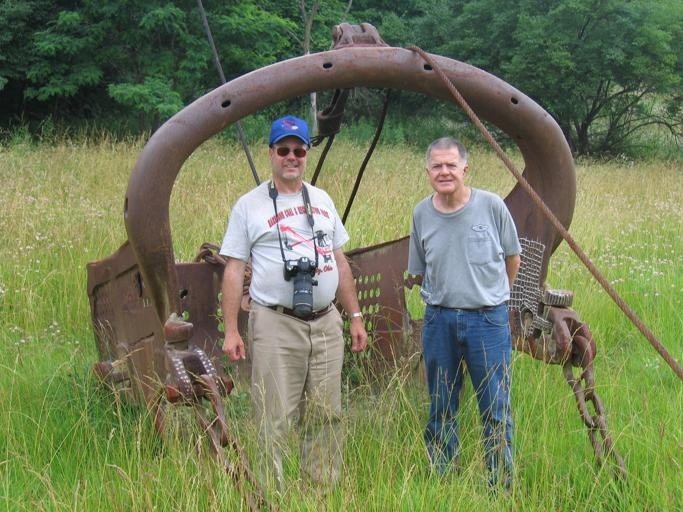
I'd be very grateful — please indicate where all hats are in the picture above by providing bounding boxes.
[268,116,310,146]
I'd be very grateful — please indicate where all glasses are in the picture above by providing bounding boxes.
[275,147,307,158]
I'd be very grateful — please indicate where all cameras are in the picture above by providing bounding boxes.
[282,259,320,316]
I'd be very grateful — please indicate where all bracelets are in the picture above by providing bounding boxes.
[347,311,364,321]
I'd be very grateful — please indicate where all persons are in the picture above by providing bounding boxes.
[217,115,369,496]
[405,136,524,502]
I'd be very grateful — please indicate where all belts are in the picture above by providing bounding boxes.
[269,303,334,321]
